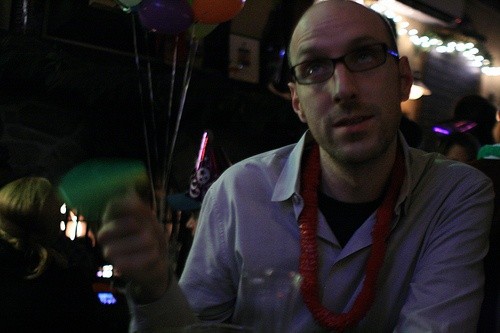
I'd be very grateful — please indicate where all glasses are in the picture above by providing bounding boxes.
[289,42,401,85]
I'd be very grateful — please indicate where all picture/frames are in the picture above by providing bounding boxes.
[226,32,264,87]
